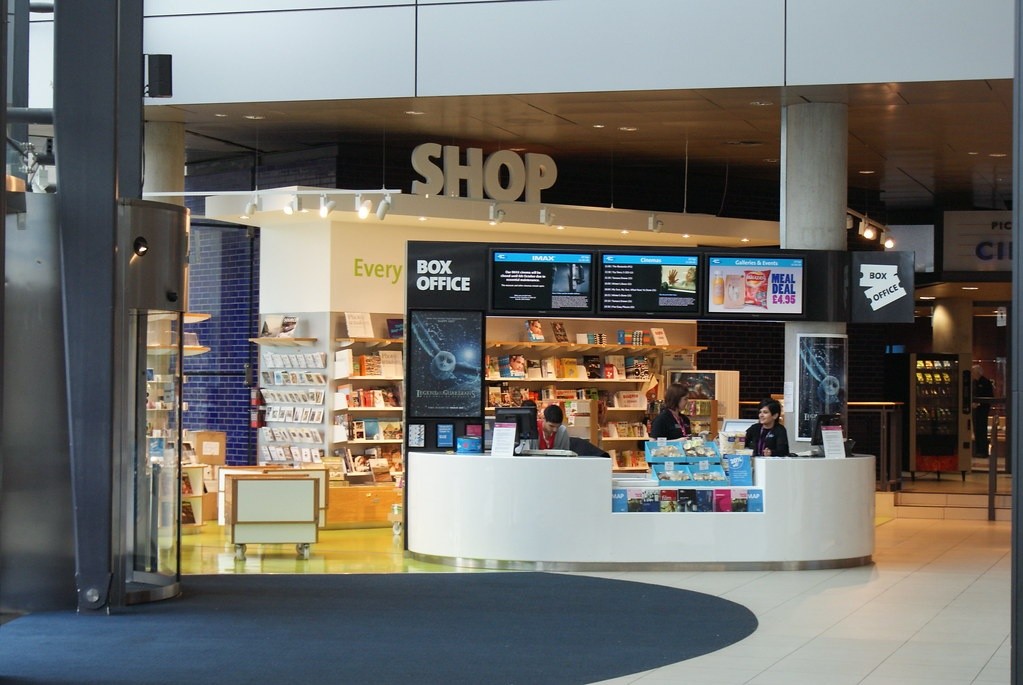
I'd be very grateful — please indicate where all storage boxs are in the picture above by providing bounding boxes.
[184,429,228,522]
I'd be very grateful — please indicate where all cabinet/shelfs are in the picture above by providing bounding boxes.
[157,463,208,534]
[326,331,406,534]
[479,313,697,474]
[666,368,741,450]
[133,309,213,529]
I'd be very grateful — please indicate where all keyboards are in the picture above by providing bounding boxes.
[521,449,578,457]
[795,449,818,457]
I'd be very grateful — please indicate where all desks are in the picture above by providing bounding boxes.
[217,463,329,544]
[219,472,319,561]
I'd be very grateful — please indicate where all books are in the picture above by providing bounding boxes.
[485,316,762,513]
[253,317,402,481]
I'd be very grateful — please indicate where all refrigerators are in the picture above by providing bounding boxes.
[909,351,972,475]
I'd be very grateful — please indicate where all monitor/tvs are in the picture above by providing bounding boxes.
[721,419,760,432]
[810,414,841,446]
[703,252,807,318]
[495,406,538,456]
[597,250,703,317]
[488,248,595,315]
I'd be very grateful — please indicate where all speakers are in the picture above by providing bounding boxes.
[148,54,172,97]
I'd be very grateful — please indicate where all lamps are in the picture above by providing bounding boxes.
[646,213,663,233]
[241,189,394,221]
[537,206,556,229]
[842,213,898,249]
[487,203,503,226]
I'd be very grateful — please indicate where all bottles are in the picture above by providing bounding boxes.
[711,269,724,305]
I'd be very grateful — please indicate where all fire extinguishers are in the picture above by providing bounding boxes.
[250,387,269,429]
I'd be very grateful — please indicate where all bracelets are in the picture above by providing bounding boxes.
[977,403,981,408]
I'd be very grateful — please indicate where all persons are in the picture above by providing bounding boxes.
[650,384,693,440]
[520,399,540,418]
[745,398,790,456]
[536,405,573,450]
[668,267,697,288]
[971,364,995,459]
[528,319,542,336]
[509,354,525,374]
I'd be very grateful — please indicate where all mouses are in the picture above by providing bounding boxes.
[787,453,798,457]
